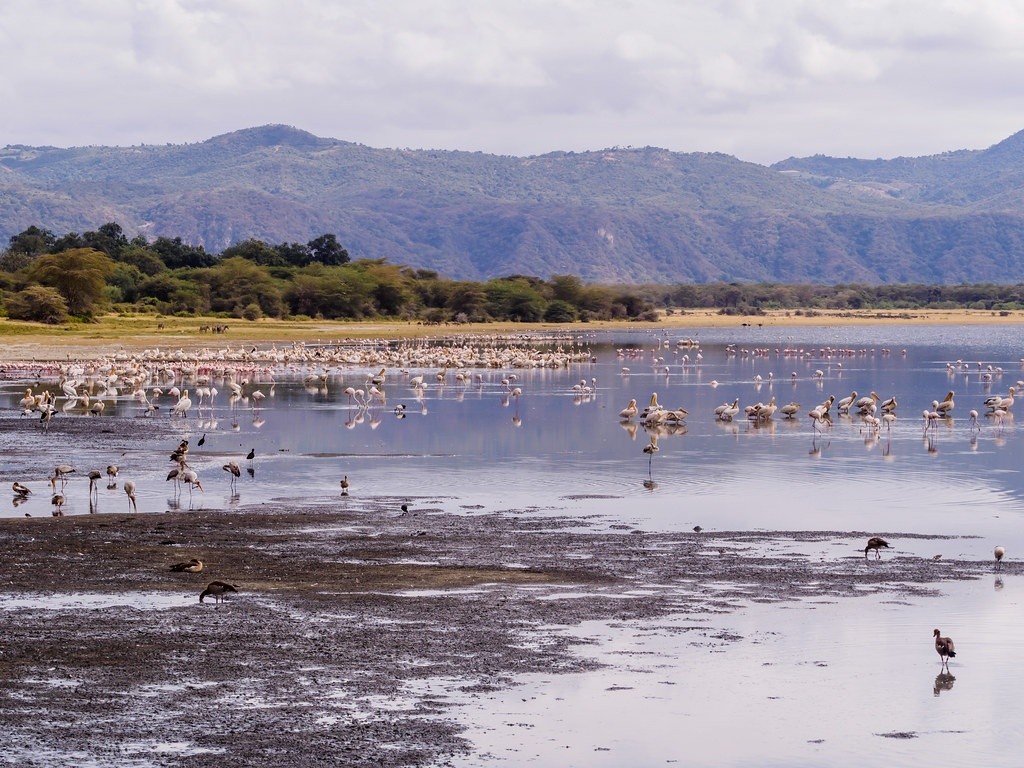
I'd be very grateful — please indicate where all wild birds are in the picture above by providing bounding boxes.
[0,330,1024,467]
[106,465,119,480]
[341,475,348,490]
[124,480,136,498]
[166,460,204,495]
[223,461,241,481]
[933,629,956,662]
[169,558,203,573]
[994,545,1004,561]
[199,581,238,604]
[88,470,102,488]
[12,482,33,495]
[52,495,64,507]
[865,538,890,559]
[52,465,76,487]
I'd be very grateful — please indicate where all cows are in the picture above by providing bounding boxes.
[200,323,229,333]
[417,320,471,326]
